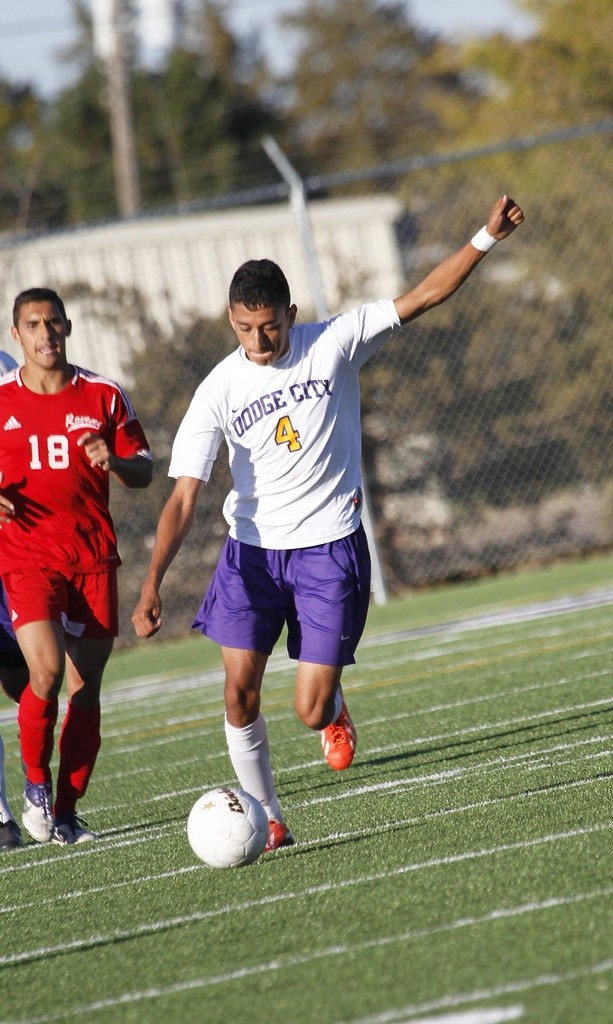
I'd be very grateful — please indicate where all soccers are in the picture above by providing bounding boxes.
[187,787,270,870]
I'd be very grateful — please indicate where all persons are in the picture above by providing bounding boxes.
[0,287,154,847]
[132,191,525,853]
[0,350,68,851]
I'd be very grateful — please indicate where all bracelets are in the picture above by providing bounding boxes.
[470,225,498,252]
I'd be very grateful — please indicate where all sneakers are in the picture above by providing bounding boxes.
[320,682,357,771]
[21,778,54,843]
[47,813,98,848]
[0,820,23,850]
[263,820,294,851]
